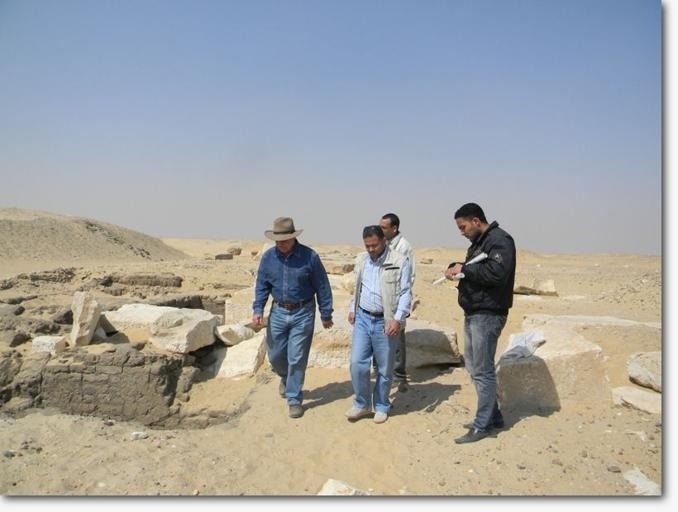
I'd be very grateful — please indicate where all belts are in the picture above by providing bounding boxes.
[358,304,384,317]
[274,296,314,310]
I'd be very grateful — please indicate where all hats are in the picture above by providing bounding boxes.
[263,216,305,242]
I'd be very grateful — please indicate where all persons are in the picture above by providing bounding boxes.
[250,217,336,420]
[442,202,518,445]
[377,213,417,394]
[344,224,413,425]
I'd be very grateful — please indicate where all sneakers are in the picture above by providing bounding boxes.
[287,403,304,418]
[372,409,389,423]
[278,377,289,398]
[345,402,373,420]
[397,378,409,393]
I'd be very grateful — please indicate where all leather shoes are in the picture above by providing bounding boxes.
[463,413,505,429]
[454,426,490,443]
